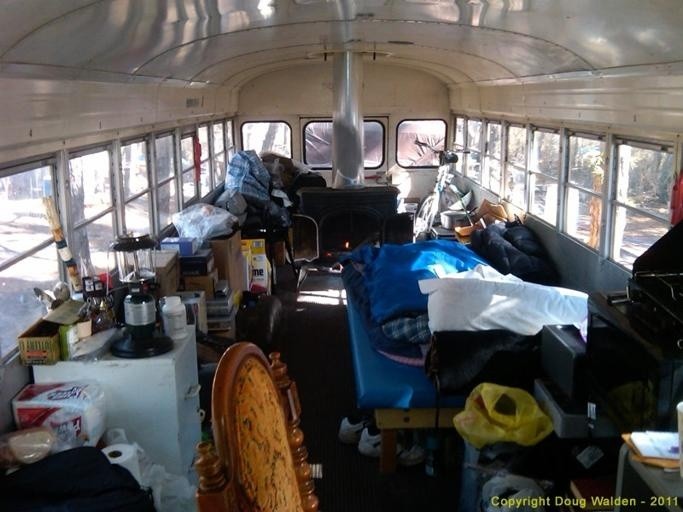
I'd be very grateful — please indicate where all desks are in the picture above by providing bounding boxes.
[614,430,682,512]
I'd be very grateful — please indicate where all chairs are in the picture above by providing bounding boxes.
[193,336,322,512]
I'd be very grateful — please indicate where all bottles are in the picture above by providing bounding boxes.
[161,296,188,340]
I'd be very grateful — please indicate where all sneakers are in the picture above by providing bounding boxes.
[357,428,425,464]
[339,417,371,444]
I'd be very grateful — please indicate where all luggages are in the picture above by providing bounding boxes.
[236,290,283,355]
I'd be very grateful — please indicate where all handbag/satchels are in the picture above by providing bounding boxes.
[224,150,270,202]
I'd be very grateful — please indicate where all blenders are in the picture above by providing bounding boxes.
[109,230,173,358]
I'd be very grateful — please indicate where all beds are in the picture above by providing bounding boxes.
[342,240,548,474]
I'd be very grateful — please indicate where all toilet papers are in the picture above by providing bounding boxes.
[99,443,143,489]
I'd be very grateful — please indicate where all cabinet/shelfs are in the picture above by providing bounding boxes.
[26,324,205,494]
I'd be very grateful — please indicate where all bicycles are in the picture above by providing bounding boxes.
[411,139,468,233]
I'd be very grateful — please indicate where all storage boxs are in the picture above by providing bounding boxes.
[144,249,181,299]
[179,247,214,275]
[204,288,233,315]
[16,318,63,367]
[162,236,198,255]
[182,269,224,300]
[206,230,242,295]
[10,380,108,447]
[205,303,237,334]
